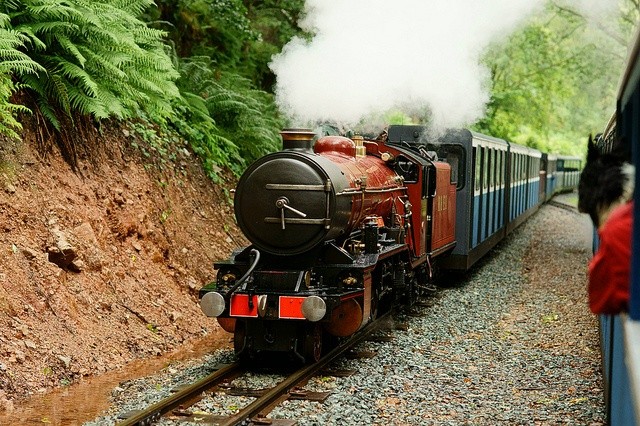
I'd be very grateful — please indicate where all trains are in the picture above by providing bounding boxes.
[579,30,640,426]
[198,125,581,371]
